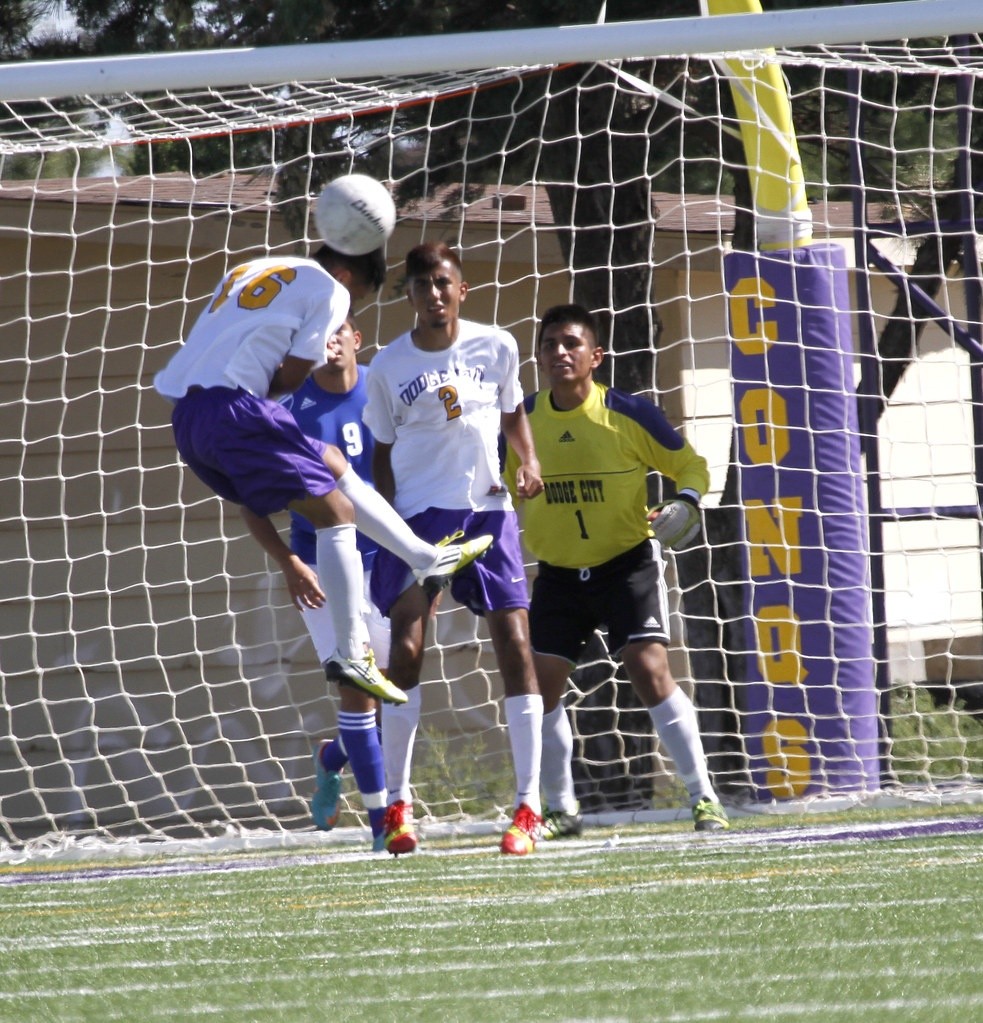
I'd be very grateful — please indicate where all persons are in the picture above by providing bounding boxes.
[361,242,545,857]
[155,246,496,705]
[497,304,730,841]
[240,308,391,852]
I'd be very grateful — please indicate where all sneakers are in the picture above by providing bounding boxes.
[412,530,493,600]
[313,739,343,830]
[325,648,408,706]
[542,801,583,839]
[692,799,730,832]
[501,803,542,857]
[385,799,416,854]
[374,830,386,851]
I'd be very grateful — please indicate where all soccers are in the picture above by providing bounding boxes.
[313,172,399,259]
